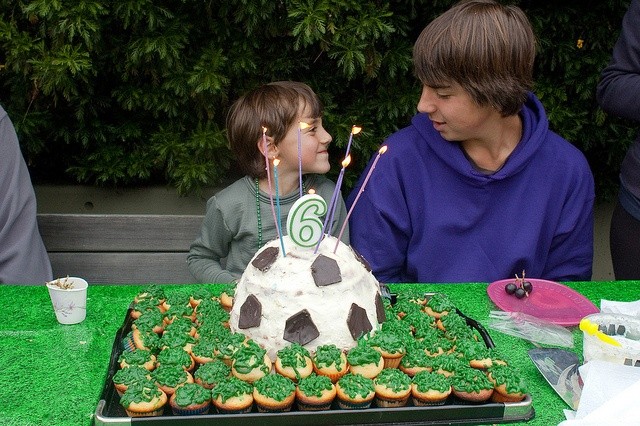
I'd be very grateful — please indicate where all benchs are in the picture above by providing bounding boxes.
[32,212,226,286]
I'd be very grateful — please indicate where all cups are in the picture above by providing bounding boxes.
[45,277,89,324]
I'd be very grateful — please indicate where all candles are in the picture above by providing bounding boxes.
[328,125,362,238]
[262,127,279,238]
[333,145,388,255]
[315,154,352,254]
[273,156,287,257]
[297,121,310,198]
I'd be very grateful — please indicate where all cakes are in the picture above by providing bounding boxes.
[194,302,230,317]
[398,349,433,377]
[401,310,437,333]
[227,232,391,362]
[117,348,157,372]
[168,382,212,416]
[437,312,468,332]
[134,291,166,306]
[190,344,218,365]
[162,298,192,311]
[122,329,160,353]
[487,366,528,402]
[312,344,351,384]
[424,294,457,319]
[153,370,195,395]
[411,370,452,406]
[160,338,196,355]
[433,353,469,378]
[131,318,168,333]
[129,304,166,320]
[453,338,487,360]
[346,330,385,380]
[162,325,196,336]
[335,372,377,410]
[193,362,233,390]
[396,286,428,306]
[211,376,253,414]
[470,355,509,370]
[120,385,169,417]
[220,287,236,312]
[393,301,424,318]
[231,339,273,384]
[372,366,412,408]
[452,366,495,403]
[381,318,413,345]
[214,335,251,367]
[381,298,401,322]
[295,371,337,411]
[366,329,407,370]
[155,354,196,377]
[195,317,231,328]
[274,342,314,383]
[111,364,153,396]
[163,311,197,325]
[194,324,231,342]
[189,288,217,309]
[253,372,295,412]
[411,331,451,357]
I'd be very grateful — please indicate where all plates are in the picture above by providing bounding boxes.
[487,278,600,326]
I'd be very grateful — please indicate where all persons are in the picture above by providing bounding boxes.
[595,1,640,280]
[0,102,53,284]
[185,80,350,284]
[344,0,594,281]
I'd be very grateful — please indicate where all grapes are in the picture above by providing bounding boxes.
[520,281,532,293]
[515,288,525,298]
[504,282,517,295]
